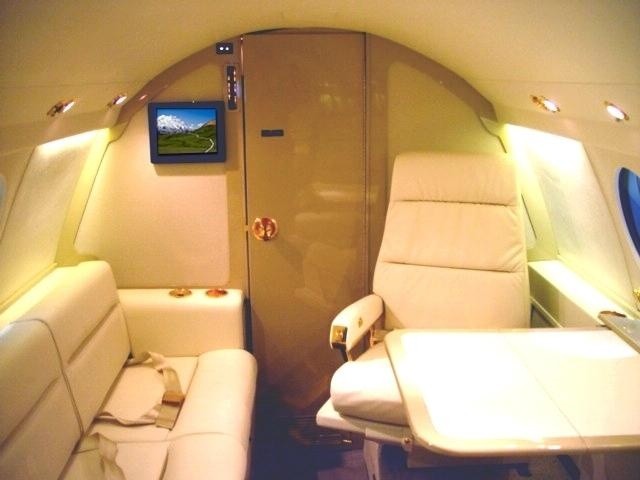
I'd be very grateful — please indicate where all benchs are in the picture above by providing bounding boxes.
[0,254,259,478]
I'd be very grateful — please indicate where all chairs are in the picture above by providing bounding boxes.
[312,142,536,480]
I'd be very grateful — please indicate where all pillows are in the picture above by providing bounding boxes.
[325,357,410,430]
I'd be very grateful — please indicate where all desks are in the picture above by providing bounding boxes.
[377,321,640,479]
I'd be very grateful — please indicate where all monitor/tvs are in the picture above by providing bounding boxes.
[147,100,225,164]
